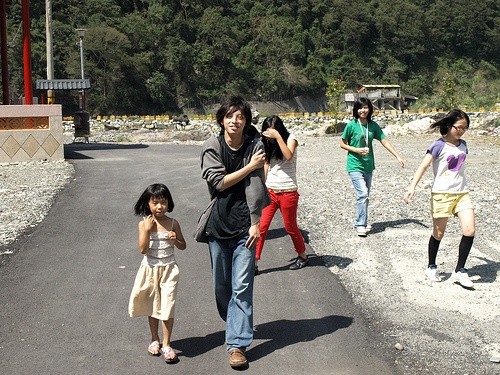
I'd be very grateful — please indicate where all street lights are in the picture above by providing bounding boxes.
[75,28,88,110]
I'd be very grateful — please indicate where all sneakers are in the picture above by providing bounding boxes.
[254,267,258,276]
[425,267,441,281]
[452,269,473,288]
[289,255,310,270]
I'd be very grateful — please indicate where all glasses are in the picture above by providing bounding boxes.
[450,123,468,132]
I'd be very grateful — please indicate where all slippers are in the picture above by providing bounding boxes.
[159,347,177,363]
[147,340,160,356]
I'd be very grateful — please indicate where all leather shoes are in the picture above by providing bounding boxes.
[229,349,247,368]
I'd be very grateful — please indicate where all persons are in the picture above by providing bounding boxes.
[339,97,404,235]
[253,115,309,276]
[127,184,187,363]
[404,109,475,288]
[194,96,272,368]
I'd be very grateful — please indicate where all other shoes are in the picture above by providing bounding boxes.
[357,225,367,237]
